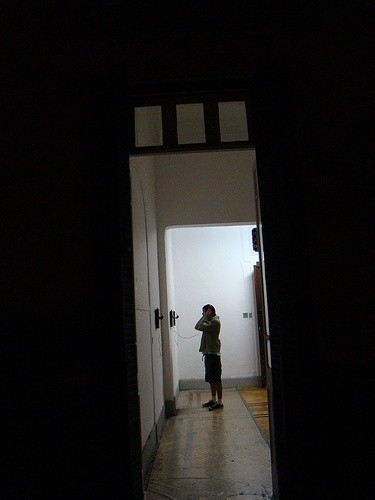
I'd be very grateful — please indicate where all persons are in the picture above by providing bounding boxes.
[195,304,224,411]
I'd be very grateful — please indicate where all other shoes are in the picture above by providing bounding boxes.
[208,403,223,410]
[201,400,217,407]
